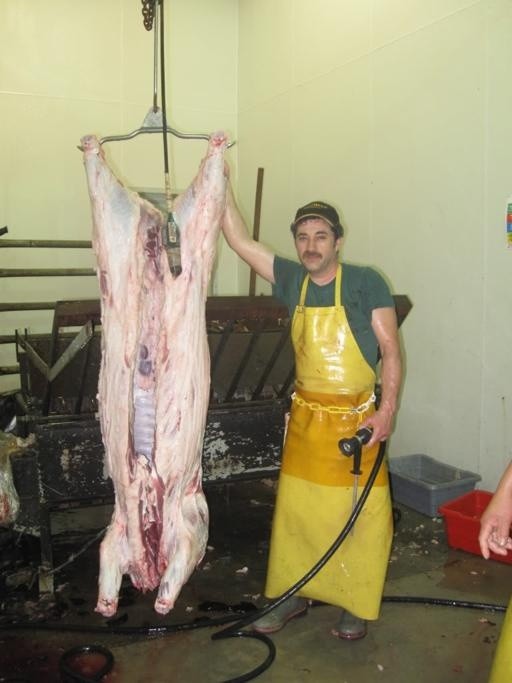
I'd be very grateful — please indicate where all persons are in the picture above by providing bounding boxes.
[220,160,404,640]
[477,457,511,683]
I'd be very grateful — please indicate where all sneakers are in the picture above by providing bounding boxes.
[251,596,307,632]
[338,609,367,639]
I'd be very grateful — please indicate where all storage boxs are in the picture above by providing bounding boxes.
[439,490,512,562]
[387,453,482,519]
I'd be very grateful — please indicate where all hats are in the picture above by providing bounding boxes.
[291,202,343,234]
